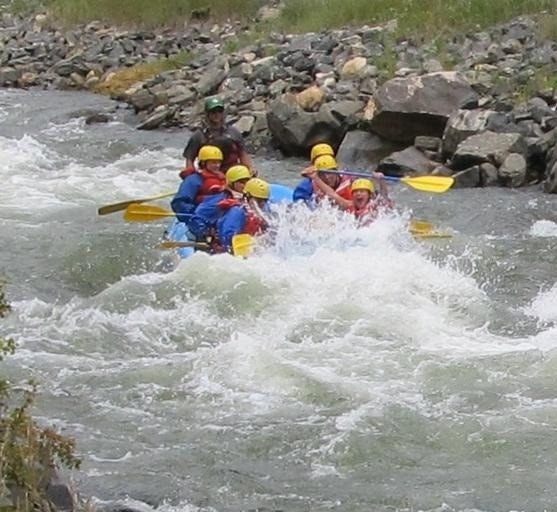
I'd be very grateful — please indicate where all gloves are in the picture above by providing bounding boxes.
[167,182,416,266]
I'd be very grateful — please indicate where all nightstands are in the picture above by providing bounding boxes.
[98,192,193,221]
[408,219,453,238]
[232,234,263,258]
[154,242,229,250]
[316,170,455,193]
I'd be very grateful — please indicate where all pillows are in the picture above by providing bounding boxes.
[223,165,271,199]
[349,175,376,197]
[196,143,224,163]
[310,142,337,173]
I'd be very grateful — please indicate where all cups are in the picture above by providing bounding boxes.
[211,109,224,114]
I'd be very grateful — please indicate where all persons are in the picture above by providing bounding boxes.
[217,178,276,254]
[190,165,252,249]
[181,96,256,177]
[293,145,336,201]
[170,145,226,234]
[312,155,358,204]
[305,166,392,215]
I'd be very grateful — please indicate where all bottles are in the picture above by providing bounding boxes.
[203,94,225,112]
